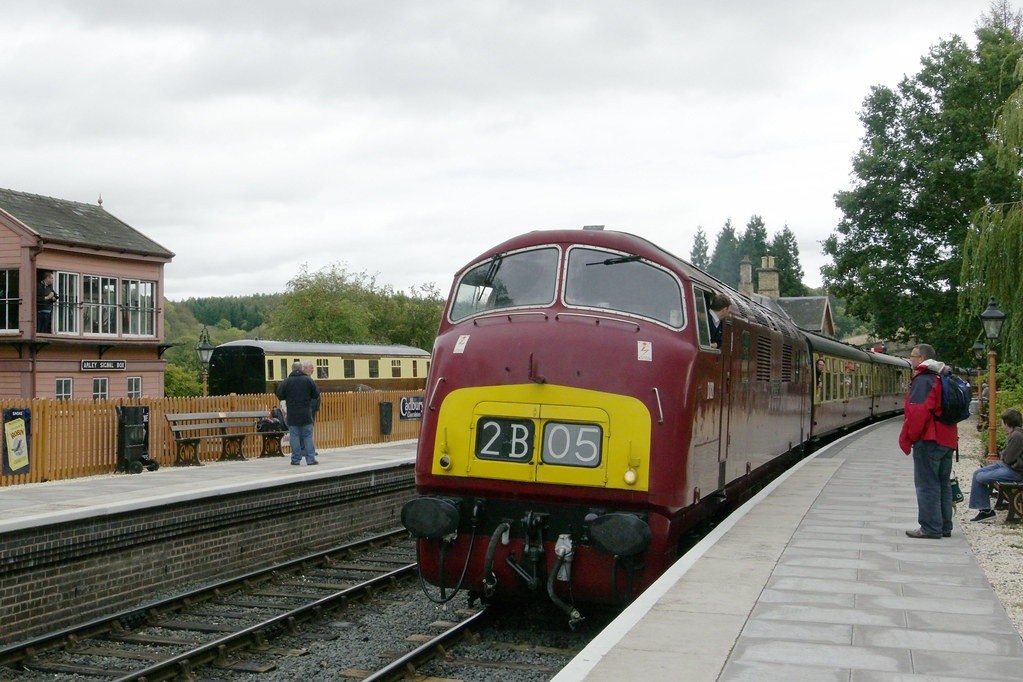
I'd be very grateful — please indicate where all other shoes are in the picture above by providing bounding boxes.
[291,461,300,465]
[942,528,952,537]
[906,530,942,539]
[308,461,318,465]
[970,508,996,522]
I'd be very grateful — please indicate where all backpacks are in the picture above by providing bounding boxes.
[921,369,971,424]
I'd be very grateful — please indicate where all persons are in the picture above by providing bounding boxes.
[276,360,321,465]
[707,294,732,349]
[817,359,826,386]
[982,383,989,398]
[899,343,958,539]
[964,377,971,391]
[969,408,1023,522]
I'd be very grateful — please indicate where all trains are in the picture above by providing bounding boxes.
[400,226,990,633]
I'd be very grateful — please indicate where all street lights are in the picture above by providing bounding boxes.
[980,291,1007,465]
[194,323,215,397]
[971,340,985,422]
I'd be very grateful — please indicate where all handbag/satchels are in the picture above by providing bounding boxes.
[270,406,287,431]
[950,479,964,503]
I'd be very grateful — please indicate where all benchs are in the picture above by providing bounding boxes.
[165,411,290,466]
[994,479,1023,526]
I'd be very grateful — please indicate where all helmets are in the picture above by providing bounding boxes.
[255,418,281,432]
